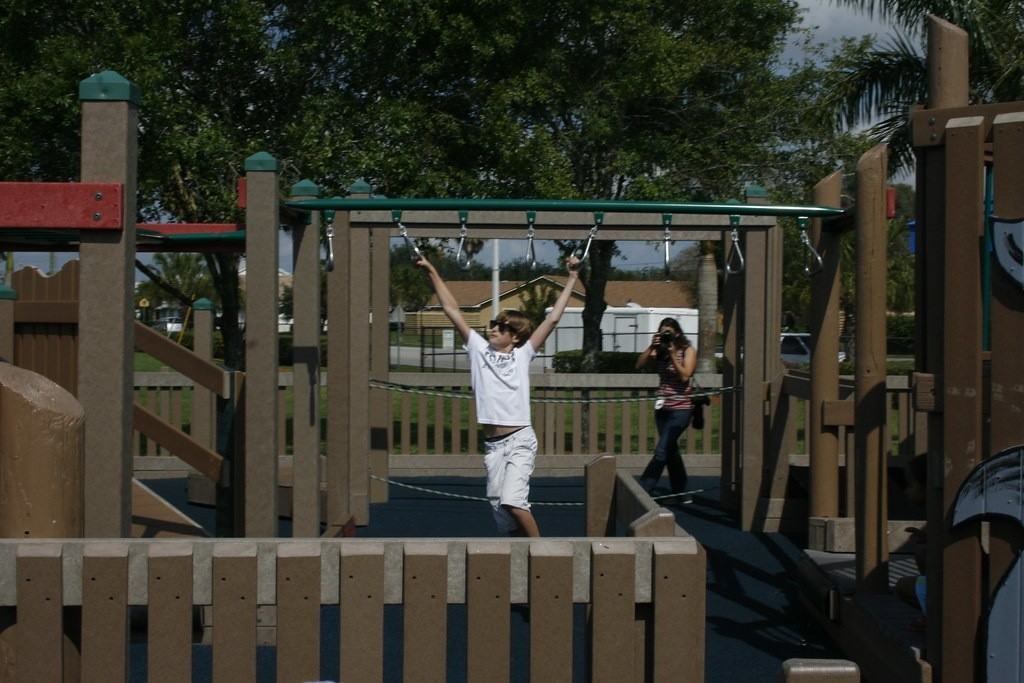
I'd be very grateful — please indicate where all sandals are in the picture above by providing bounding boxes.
[906,617,927,632]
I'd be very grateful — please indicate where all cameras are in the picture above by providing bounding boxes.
[658,331,673,344]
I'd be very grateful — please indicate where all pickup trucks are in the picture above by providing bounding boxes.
[713,332,847,365]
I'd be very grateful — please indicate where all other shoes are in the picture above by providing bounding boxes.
[661,489,694,505]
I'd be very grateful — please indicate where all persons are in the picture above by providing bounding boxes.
[892,453,937,632]
[634,317,697,510]
[411,253,583,537]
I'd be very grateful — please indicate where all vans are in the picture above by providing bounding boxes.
[155,317,182,333]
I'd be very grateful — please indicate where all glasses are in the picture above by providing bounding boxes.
[489,320,516,332]
